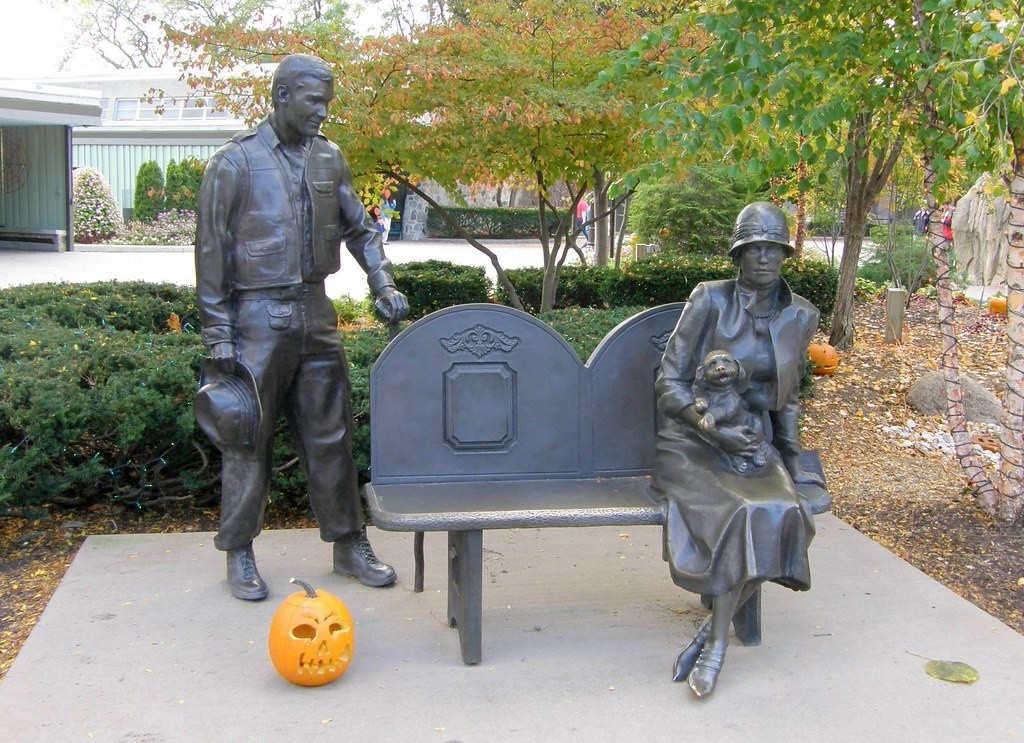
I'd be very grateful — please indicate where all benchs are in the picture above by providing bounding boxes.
[364,303,832,668]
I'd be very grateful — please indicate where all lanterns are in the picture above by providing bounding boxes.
[268,577,356,687]
[806,337,838,376]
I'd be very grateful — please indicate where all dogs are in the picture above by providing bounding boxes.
[688,351,770,472]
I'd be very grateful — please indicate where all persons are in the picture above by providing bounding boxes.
[375,186,397,245]
[191,52,411,600]
[913,200,956,238]
[576,195,595,240]
[653,201,826,697]
